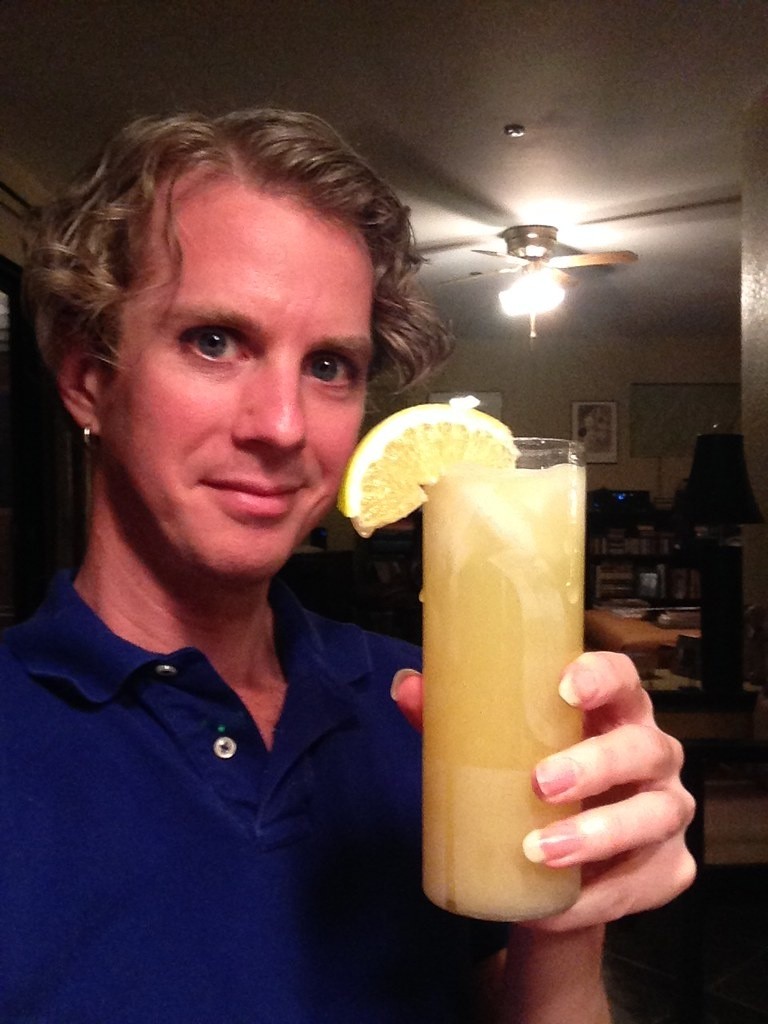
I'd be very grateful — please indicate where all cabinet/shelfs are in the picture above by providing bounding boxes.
[585,509,700,610]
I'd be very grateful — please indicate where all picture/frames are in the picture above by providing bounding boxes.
[570,399,619,465]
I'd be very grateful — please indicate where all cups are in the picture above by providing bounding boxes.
[420,435,584,921]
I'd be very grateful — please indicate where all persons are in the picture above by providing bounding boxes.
[0,108,698,1024]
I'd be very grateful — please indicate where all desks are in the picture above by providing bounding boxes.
[653,699,768,859]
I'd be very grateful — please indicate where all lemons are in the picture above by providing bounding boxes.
[337,402,519,538]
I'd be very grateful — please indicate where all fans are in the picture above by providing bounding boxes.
[436,222,639,289]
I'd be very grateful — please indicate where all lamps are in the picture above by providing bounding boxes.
[670,433,765,694]
